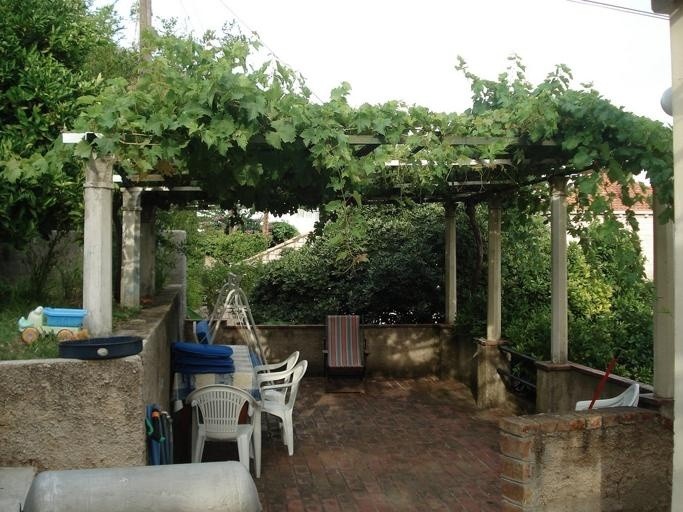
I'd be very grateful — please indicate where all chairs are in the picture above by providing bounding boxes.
[254,350,300,427]
[255,360,308,455]
[191,320,211,345]
[575,383,640,411]
[185,383,262,479]
[322,314,369,394]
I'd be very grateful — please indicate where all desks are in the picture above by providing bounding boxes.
[172,343,258,429]
